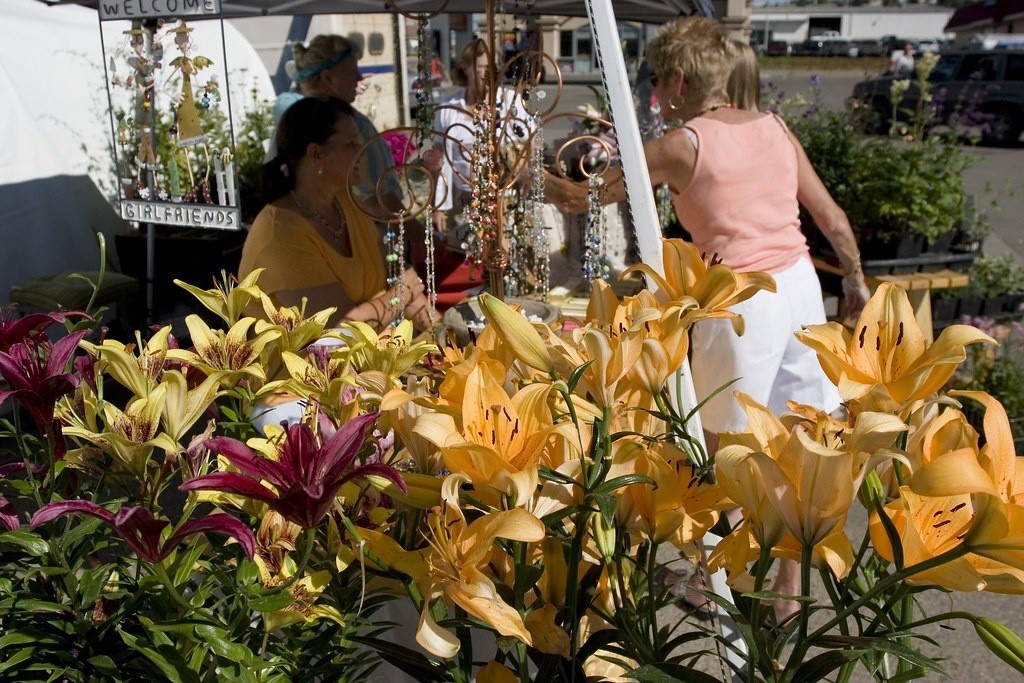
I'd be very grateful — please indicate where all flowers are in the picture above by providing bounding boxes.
[0,232,1024,683]
[758,50,1016,245]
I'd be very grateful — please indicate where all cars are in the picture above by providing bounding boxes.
[787,37,914,58]
[406,69,441,108]
[750,39,786,55]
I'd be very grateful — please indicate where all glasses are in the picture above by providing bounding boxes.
[649,67,676,87]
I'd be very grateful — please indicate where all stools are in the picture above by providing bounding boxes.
[10,268,138,342]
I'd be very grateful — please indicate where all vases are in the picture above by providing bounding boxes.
[948,236,983,273]
[865,235,924,276]
[931,295,957,321]
[1008,289,1024,314]
[985,294,1008,316]
[920,231,956,272]
[958,295,986,320]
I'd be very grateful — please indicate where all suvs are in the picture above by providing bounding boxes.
[847,46,1024,144]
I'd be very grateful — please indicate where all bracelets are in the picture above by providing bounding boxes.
[841,261,861,277]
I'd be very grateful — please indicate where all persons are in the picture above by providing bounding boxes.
[895,46,918,71]
[234,16,871,625]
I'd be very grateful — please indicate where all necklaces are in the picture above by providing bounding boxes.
[292,189,345,247]
